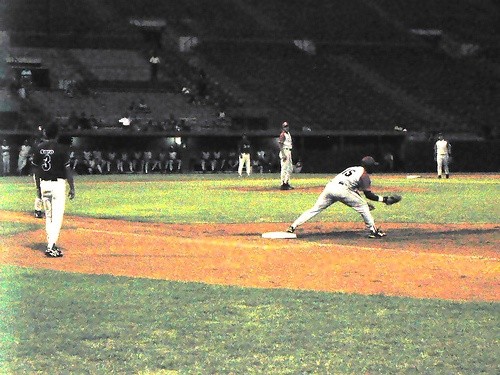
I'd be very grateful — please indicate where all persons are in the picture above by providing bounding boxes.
[132,149,145,171]
[167,146,183,173]
[149,55,161,74]
[393,121,406,131]
[33,125,75,257]
[253,144,273,172]
[92,147,106,175]
[66,151,78,172]
[433,132,453,179]
[286,155,401,239]
[277,122,293,190]
[200,147,215,174]
[237,133,251,177]
[106,147,121,174]
[143,149,158,174]
[301,121,313,131]
[69,99,224,129]
[0,139,10,173]
[226,148,239,172]
[16,140,31,175]
[211,147,226,173]
[82,148,94,175]
[120,148,133,173]
[157,148,169,173]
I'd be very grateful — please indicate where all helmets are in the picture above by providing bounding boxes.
[361,156,379,171]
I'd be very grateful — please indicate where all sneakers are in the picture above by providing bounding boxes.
[287,226,295,233]
[287,183,294,189]
[45,248,63,257]
[53,246,61,254]
[35,210,42,218]
[368,226,386,238]
[281,183,292,190]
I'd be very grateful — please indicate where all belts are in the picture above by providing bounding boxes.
[339,181,346,185]
[283,148,293,151]
[41,177,56,181]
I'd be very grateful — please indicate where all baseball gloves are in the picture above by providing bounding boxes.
[386,195,402,205]
